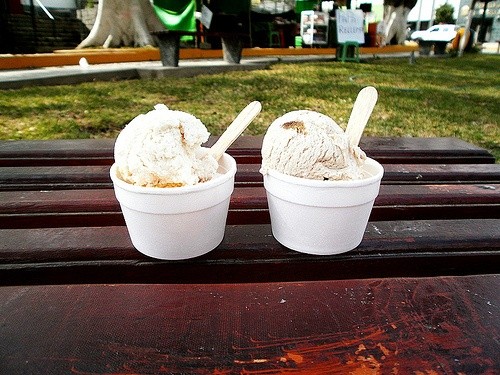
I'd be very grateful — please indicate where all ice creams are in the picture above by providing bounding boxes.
[260,109,367,181]
[114,104,225,188]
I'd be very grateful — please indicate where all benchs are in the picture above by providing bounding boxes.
[0,135,500,287]
[148,30,244,67]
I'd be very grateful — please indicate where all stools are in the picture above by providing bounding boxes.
[338,41,360,64]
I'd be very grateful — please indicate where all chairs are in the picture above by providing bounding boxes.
[268,23,280,47]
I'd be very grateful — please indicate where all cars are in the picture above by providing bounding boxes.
[411,24,461,45]
